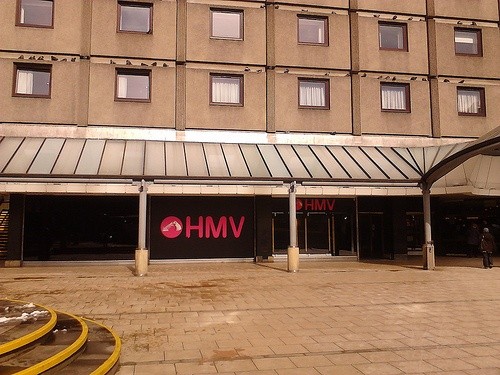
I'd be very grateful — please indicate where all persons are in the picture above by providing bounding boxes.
[463,218,482,257]
[480,227,495,270]
